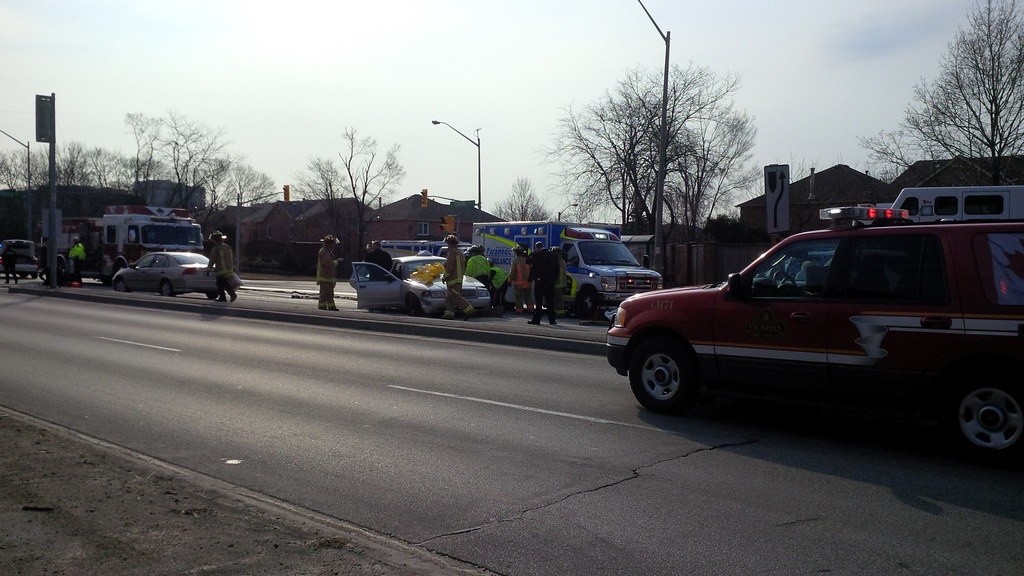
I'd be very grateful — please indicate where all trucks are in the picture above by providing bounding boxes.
[37,205,204,287]
[380,221,663,318]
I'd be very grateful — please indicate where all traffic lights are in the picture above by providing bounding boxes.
[283,185,289,204]
[421,189,427,207]
[439,216,455,232]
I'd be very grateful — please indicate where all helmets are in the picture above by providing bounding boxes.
[445,234,457,242]
[324,235,337,243]
[548,246,560,255]
[468,245,478,253]
[211,231,224,241]
[514,246,525,253]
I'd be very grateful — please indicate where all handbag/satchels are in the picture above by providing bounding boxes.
[225,272,243,290]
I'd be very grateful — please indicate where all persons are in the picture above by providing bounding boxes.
[2,243,17,284]
[206,232,237,303]
[68,237,86,288]
[316,235,340,311]
[442,235,476,321]
[507,245,536,315]
[525,241,560,325]
[548,245,577,321]
[39,267,66,286]
[363,240,393,314]
[463,245,512,318]
[794,260,813,281]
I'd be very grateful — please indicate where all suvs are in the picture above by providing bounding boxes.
[605,186,1024,454]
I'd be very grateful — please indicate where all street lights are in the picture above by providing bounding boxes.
[432,120,481,222]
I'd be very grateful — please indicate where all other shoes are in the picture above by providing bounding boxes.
[328,306,338,310]
[229,292,237,302]
[319,306,326,309]
[464,308,475,321]
[548,320,557,325]
[214,295,227,301]
[527,321,539,325]
[441,313,454,319]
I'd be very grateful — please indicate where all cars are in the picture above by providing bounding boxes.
[112,252,226,299]
[0,240,38,278]
[351,250,491,317]
